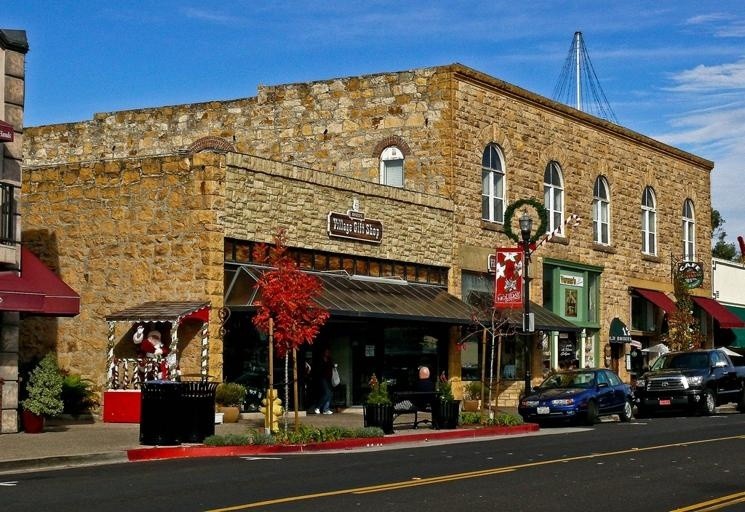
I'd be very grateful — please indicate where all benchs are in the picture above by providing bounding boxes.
[391,390,444,428]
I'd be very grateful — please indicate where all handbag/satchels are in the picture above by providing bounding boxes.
[331,367,340,387]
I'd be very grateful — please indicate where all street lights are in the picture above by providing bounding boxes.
[517,208,534,400]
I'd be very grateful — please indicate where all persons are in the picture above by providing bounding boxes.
[314,348,338,415]
[133,325,170,380]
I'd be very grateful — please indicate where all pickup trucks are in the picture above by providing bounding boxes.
[635,348,745,416]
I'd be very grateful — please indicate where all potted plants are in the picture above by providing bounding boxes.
[215,382,247,422]
[432,372,464,435]
[19,347,65,433]
[362,373,397,436]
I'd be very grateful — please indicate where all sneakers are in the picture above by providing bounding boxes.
[315,408,333,415]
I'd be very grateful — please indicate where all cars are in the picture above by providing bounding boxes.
[461,362,499,383]
[519,367,634,426]
[364,352,439,391]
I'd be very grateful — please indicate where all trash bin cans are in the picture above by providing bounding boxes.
[175,381,222,443]
[137,379,185,446]
